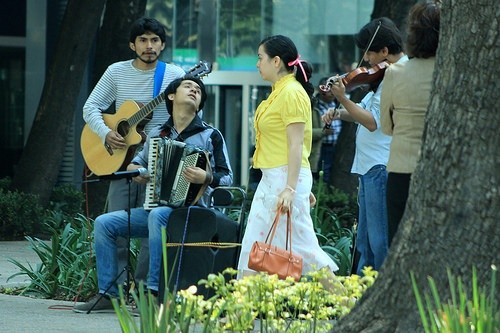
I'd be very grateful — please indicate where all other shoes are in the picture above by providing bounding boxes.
[73,295,122,307]
[129,308,141,317]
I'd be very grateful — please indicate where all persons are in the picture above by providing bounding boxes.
[312,77,342,185]
[236,35,357,303]
[73,77,233,313]
[83,16,203,283]
[322,17,410,277]
[381,0,442,248]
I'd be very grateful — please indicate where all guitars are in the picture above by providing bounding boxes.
[79,59,215,175]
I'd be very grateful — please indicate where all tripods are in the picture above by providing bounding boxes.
[86,178,140,314]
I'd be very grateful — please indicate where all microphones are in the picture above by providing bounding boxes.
[112,167,147,180]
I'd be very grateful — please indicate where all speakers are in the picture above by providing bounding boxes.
[157,206,240,318]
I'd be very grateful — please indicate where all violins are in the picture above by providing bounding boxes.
[315,59,390,98]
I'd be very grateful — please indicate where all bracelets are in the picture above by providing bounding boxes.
[286,184,296,193]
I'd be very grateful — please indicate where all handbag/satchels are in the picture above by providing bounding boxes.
[248,205,303,281]
